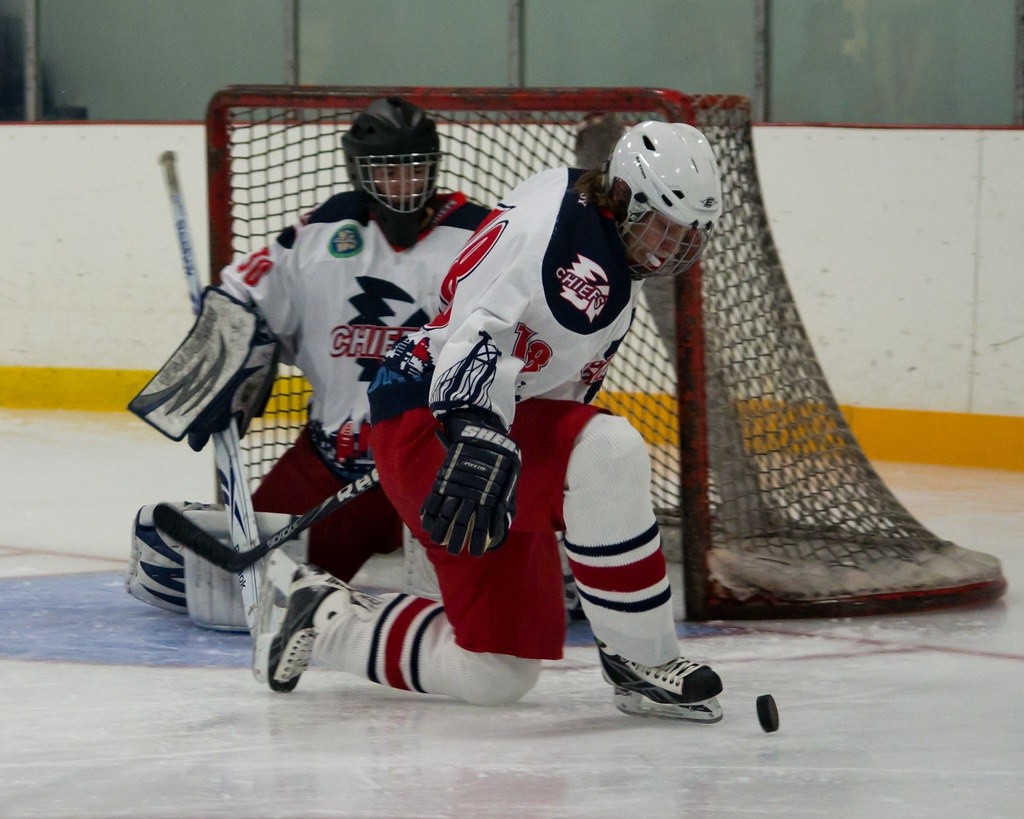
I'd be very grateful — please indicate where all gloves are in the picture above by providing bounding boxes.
[420,416,523,556]
[126,289,275,452]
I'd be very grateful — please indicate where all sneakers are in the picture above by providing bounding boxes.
[597,642,726,723]
[252,551,355,694]
[560,575,589,621]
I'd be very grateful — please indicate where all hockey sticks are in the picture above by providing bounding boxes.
[154,149,274,642]
[156,467,386,571]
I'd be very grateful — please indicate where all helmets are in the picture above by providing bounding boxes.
[342,95,441,190]
[598,119,724,237]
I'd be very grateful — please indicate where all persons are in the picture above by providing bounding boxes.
[247,118,728,723]
[121,90,496,635]
